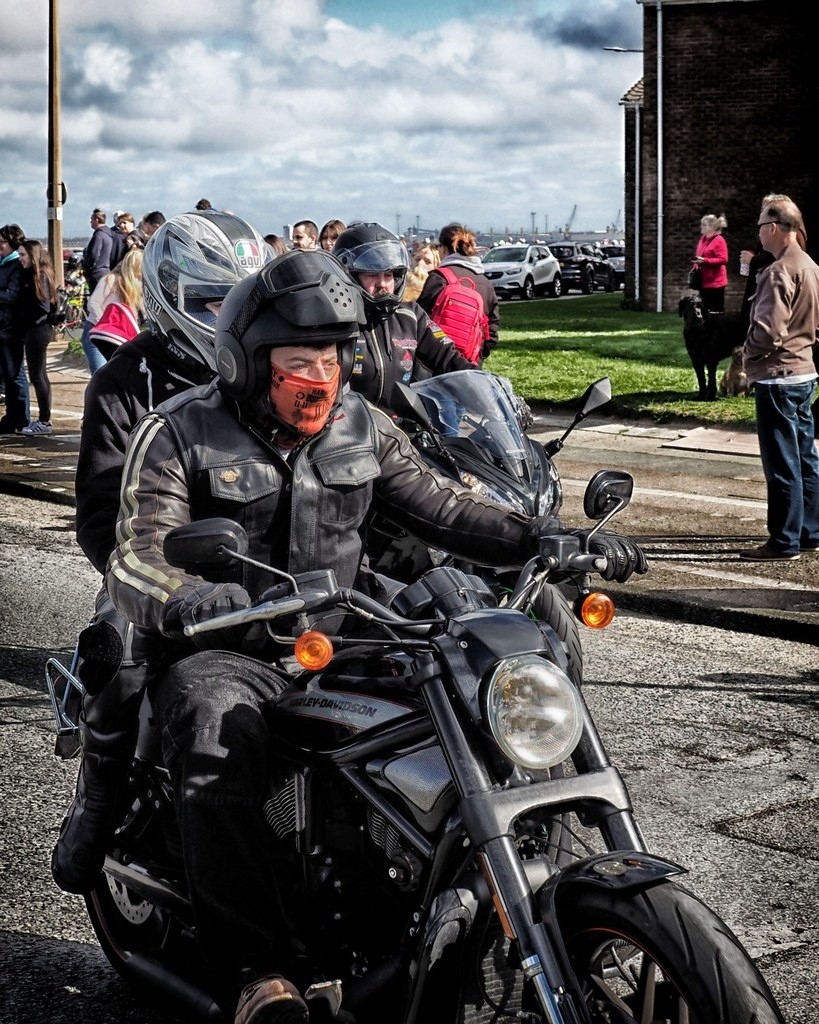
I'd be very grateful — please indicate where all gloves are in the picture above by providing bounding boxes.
[162,579,252,648]
[516,516,646,584]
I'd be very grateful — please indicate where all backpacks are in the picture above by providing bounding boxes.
[427,267,490,366]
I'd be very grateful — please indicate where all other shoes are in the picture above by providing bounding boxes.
[0,422,26,435]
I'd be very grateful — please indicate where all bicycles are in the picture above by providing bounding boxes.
[53,266,88,342]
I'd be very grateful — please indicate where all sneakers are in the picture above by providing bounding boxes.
[21,420,53,435]
[233,963,310,1024]
[740,544,800,560]
[800,543,819,551]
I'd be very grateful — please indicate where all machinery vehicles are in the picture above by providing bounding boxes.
[564,204,578,232]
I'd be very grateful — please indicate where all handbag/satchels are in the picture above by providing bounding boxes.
[687,268,702,290]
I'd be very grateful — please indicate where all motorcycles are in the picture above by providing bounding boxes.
[45,377,788,1024]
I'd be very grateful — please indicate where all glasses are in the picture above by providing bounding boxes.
[256,249,364,299]
[435,244,443,250]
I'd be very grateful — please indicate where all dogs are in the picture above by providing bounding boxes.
[719,346,749,399]
[676,288,752,401]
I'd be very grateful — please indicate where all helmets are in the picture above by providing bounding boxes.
[332,222,407,322]
[143,209,277,372]
[214,271,360,405]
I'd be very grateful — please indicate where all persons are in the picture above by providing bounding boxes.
[417,226,499,373]
[325,224,531,509]
[0,226,31,435]
[740,194,819,562]
[82,250,146,377]
[17,241,55,435]
[491,236,555,251]
[105,250,648,1024]
[691,214,728,317]
[261,218,472,312]
[75,199,220,320]
[51,210,291,888]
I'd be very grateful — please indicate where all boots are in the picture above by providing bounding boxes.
[51,710,140,894]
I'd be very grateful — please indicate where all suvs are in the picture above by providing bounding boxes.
[479,241,628,299]
